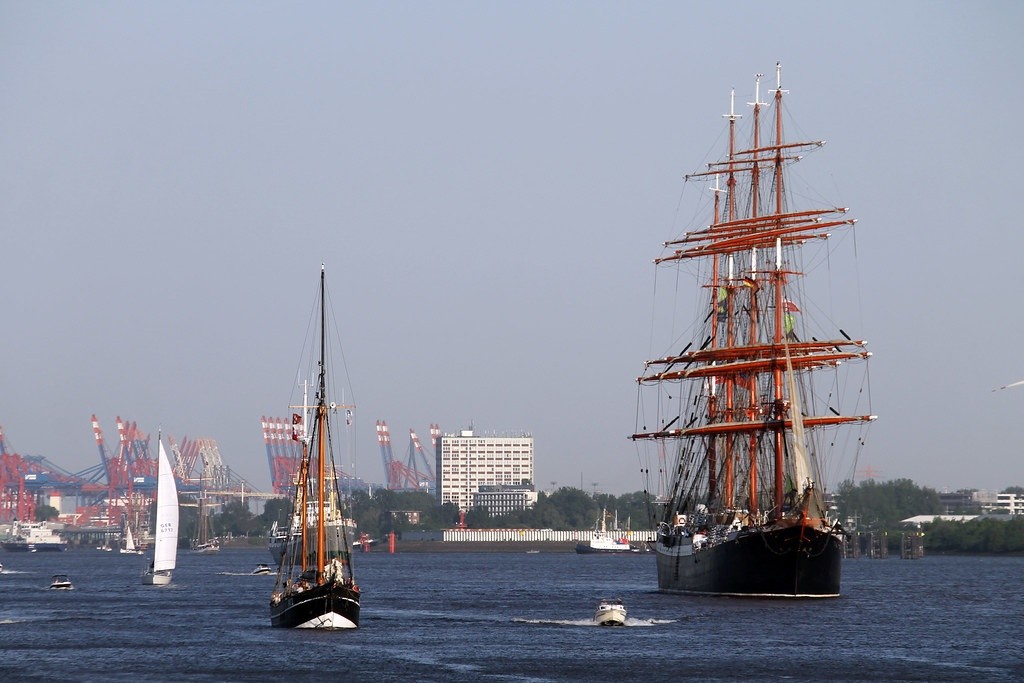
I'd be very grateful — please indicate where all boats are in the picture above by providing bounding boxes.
[594,597,626,627]
[49,574,75,591]
[252,565,271,575]
[1,517,70,553]
[270,263,361,632]
[574,508,630,553]
[628,62,880,595]
[190,490,220,554]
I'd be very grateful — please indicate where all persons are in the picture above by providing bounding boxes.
[601,599,622,605]
[293,581,305,593]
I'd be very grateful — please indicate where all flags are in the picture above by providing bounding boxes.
[783,301,799,312]
[786,314,795,339]
[292,427,299,441]
[717,287,727,322]
[293,414,302,424]
[744,276,756,287]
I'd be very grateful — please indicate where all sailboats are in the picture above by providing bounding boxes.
[120,526,144,556]
[141,426,179,585]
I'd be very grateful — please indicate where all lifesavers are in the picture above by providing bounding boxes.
[291,584,298,596]
[678,518,686,524]
